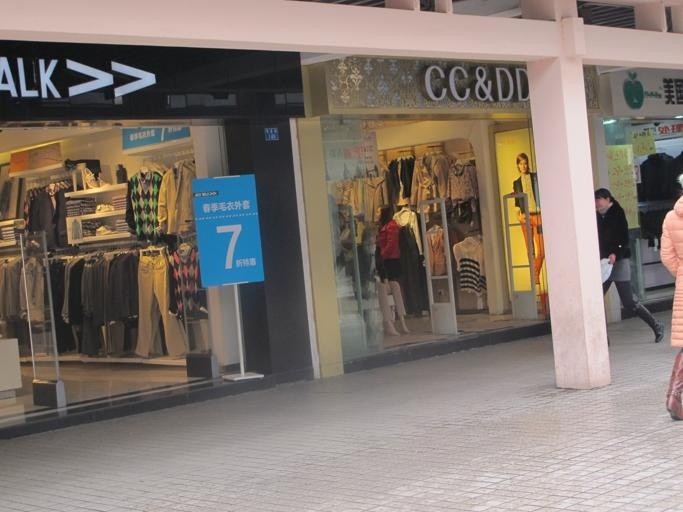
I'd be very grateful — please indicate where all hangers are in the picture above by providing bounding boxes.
[428,220,482,246]
[393,205,417,219]
[389,150,474,171]
[19,172,74,202]
[2,245,199,276]
[129,156,195,183]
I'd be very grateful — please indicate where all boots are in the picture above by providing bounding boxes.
[636,305,664,342]
[667,352,683,420]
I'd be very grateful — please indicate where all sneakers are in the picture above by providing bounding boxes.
[96,204,112,212]
[97,179,110,187]
[84,168,99,188]
[96,225,112,236]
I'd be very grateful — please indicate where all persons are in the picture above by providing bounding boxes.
[595,188,664,342]
[375,206,401,280]
[512,153,545,292]
[352,230,410,336]
[660,175,683,421]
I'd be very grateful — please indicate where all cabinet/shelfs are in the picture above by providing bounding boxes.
[60,183,133,248]
